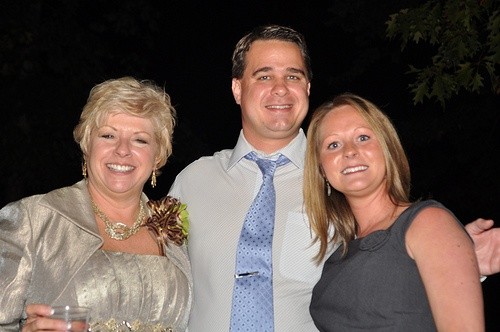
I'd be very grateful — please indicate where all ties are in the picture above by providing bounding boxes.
[229,151,290,332]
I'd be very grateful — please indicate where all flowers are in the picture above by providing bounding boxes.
[141,195,191,246]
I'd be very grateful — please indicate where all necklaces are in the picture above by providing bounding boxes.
[89,196,146,241]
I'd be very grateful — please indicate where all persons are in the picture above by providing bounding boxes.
[302,95,485,332]
[165,26,500,332]
[0,76,195,332]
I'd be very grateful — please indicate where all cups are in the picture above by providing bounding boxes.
[48,306,91,332]
